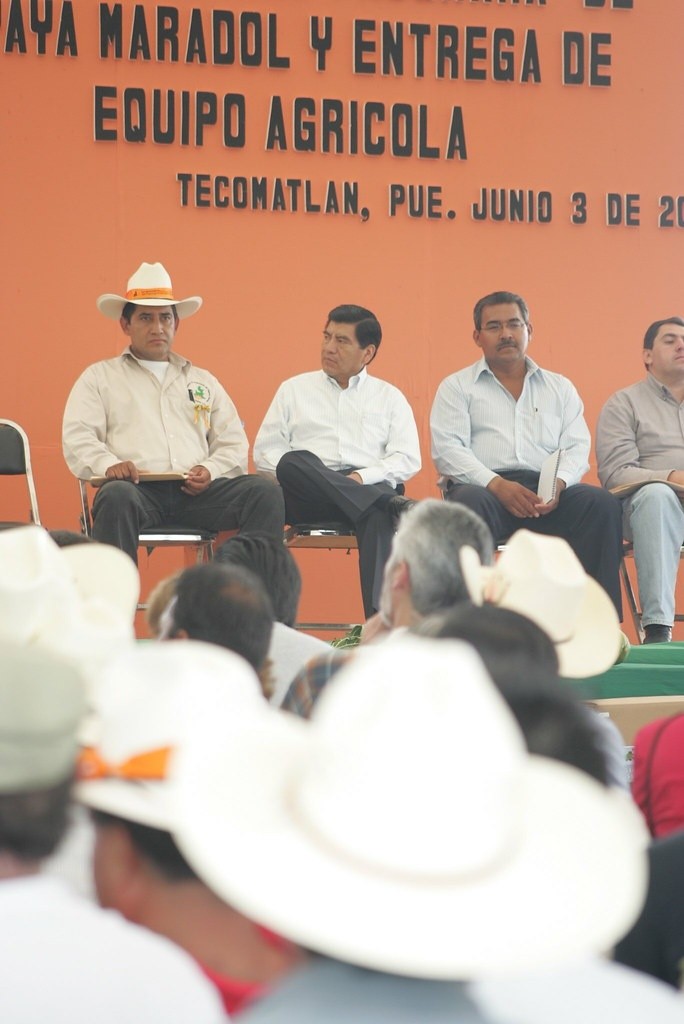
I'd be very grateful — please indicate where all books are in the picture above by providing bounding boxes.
[91,472,188,487]
[537,448,561,504]
[609,479,684,496]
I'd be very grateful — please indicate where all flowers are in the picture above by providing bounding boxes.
[193,401,212,429]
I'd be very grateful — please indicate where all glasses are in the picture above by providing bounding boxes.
[481,322,525,334]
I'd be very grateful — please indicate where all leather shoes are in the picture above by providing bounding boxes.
[389,494,421,532]
[644,624,672,644]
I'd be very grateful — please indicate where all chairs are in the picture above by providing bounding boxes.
[283,523,366,631]
[78,478,218,611]
[439,473,510,552]
[0,418,41,530]
[619,540,684,643]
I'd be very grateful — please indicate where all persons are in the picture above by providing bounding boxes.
[253,305,421,619]
[430,290,623,626]
[1,494,684,1024]
[61,262,286,568]
[595,318,684,644]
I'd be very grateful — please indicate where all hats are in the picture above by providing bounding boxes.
[483,525,621,678]
[166,635,649,979]
[97,262,202,321]
[66,637,266,830]
[0,633,93,792]
[0,524,140,666]
